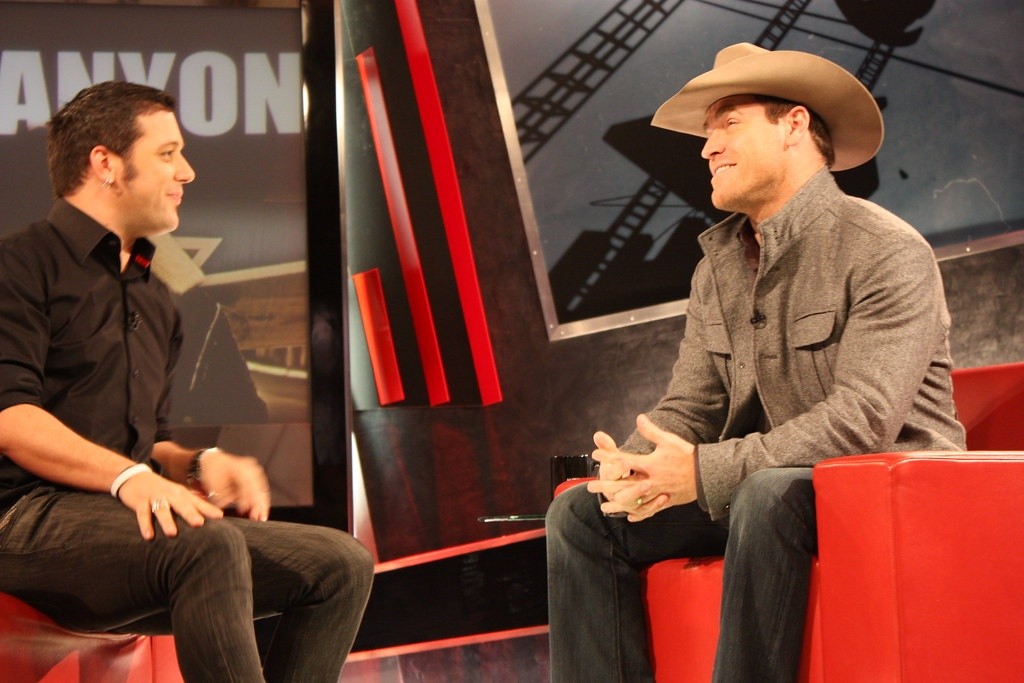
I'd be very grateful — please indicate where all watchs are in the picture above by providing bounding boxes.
[184,446,223,499]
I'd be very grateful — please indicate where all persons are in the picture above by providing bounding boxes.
[545,42,967,683]
[0,80,374,683]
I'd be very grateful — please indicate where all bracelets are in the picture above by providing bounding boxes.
[110,462,152,501]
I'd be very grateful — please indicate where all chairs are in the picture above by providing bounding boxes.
[554,362,1024,683]
[0,480,206,683]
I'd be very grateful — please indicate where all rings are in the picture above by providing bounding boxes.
[151,497,168,513]
[637,499,642,506]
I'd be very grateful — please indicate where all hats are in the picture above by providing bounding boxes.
[650,43,884,172]
